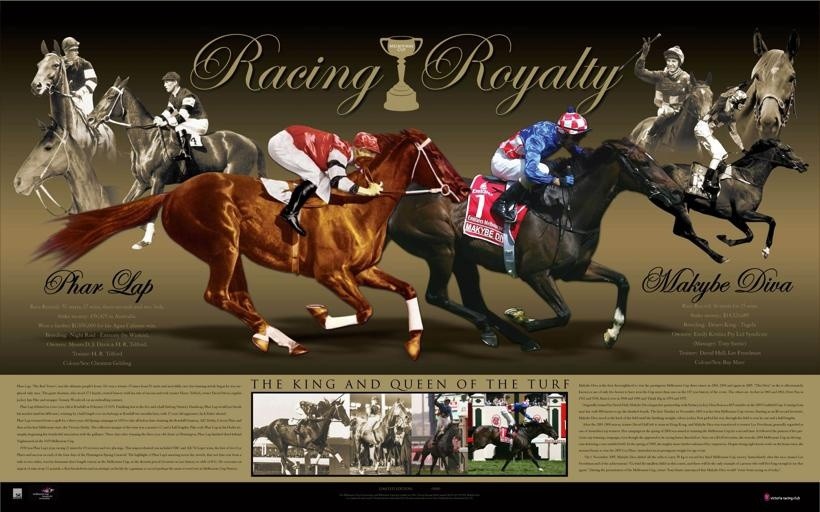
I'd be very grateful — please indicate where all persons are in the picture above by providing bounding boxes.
[694,82,748,192]
[288,401,325,434]
[153,72,209,161]
[267,125,384,236]
[361,397,381,431]
[491,106,588,221]
[634,37,690,136]
[501,401,534,437]
[61,37,97,99]
[432,393,452,441]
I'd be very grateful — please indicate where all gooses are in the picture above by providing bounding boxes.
[380,36,423,111]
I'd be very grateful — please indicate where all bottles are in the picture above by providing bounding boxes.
[62,36,80,50]
[162,72,180,81]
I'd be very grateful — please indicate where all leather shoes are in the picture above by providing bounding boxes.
[356,181,383,197]
[738,80,747,88]
[559,174,574,187]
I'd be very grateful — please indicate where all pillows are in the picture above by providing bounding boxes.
[730,87,746,109]
[352,132,381,156]
[557,107,591,135]
[663,46,685,64]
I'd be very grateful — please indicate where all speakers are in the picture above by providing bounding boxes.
[702,168,720,189]
[172,129,192,160]
[492,181,529,221]
[280,180,317,235]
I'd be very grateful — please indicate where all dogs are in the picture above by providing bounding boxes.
[629,70,714,155]
[732,31,801,142]
[414,421,461,475]
[12,113,120,215]
[252,399,350,475]
[386,136,684,354]
[86,75,267,251]
[469,417,558,472]
[660,137,809,265]
[31,40,119,169]
[23,127,471,363]
[350,399,410,476]
